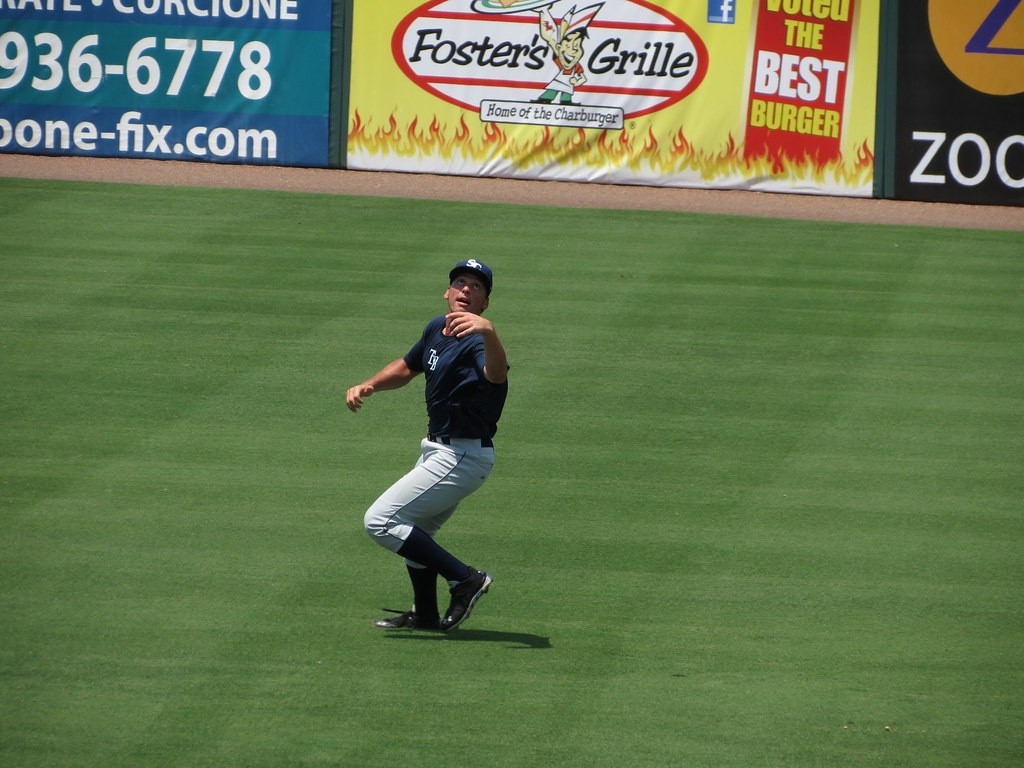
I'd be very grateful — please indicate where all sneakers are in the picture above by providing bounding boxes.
[376,608,440,629]
[440,566,494,633]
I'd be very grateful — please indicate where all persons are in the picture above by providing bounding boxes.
[344,258,511,631]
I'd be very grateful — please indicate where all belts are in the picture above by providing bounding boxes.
[426,433,494,447]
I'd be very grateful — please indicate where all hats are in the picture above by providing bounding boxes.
[449,259,493,286]
